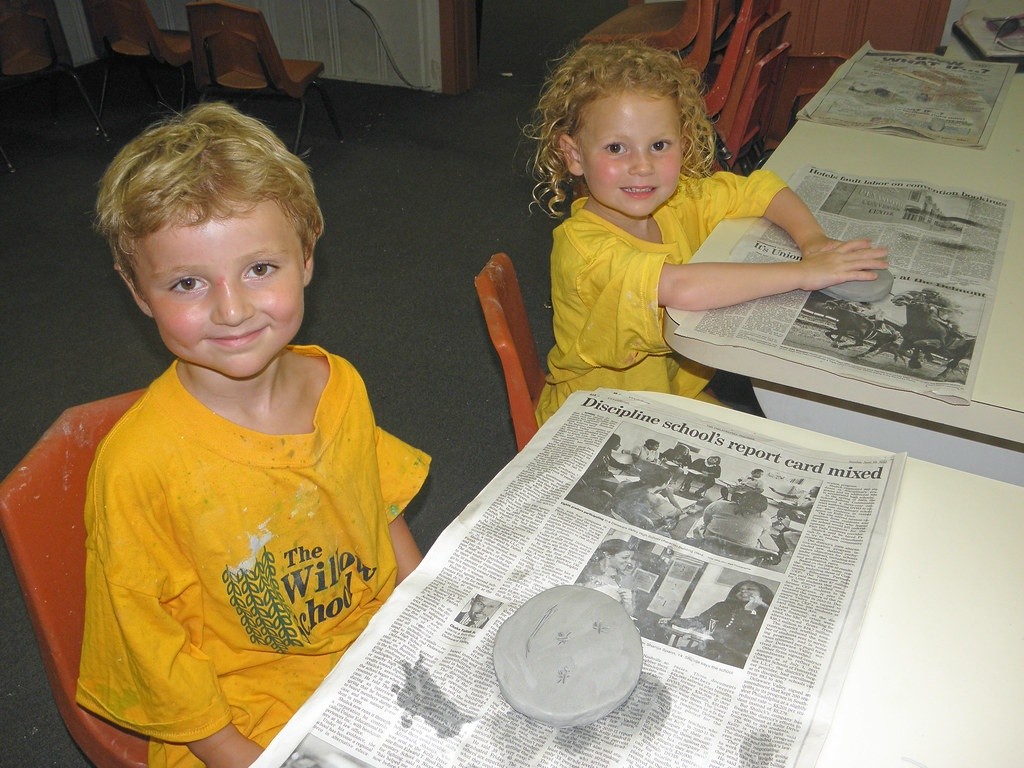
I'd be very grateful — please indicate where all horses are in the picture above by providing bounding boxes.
[821,290,976,382]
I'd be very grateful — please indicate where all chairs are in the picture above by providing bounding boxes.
[476,252,547,451]
[84,0,193,135]
[581,0,793,177]
[0,1,113,173]
[185,0,344,156]
[2,385,152,768]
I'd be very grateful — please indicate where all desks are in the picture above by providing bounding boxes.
[248,386,1023,768]
[665,41,1024,484]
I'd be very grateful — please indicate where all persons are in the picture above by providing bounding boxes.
[523,37,888,433]
[74,102,432,768]
